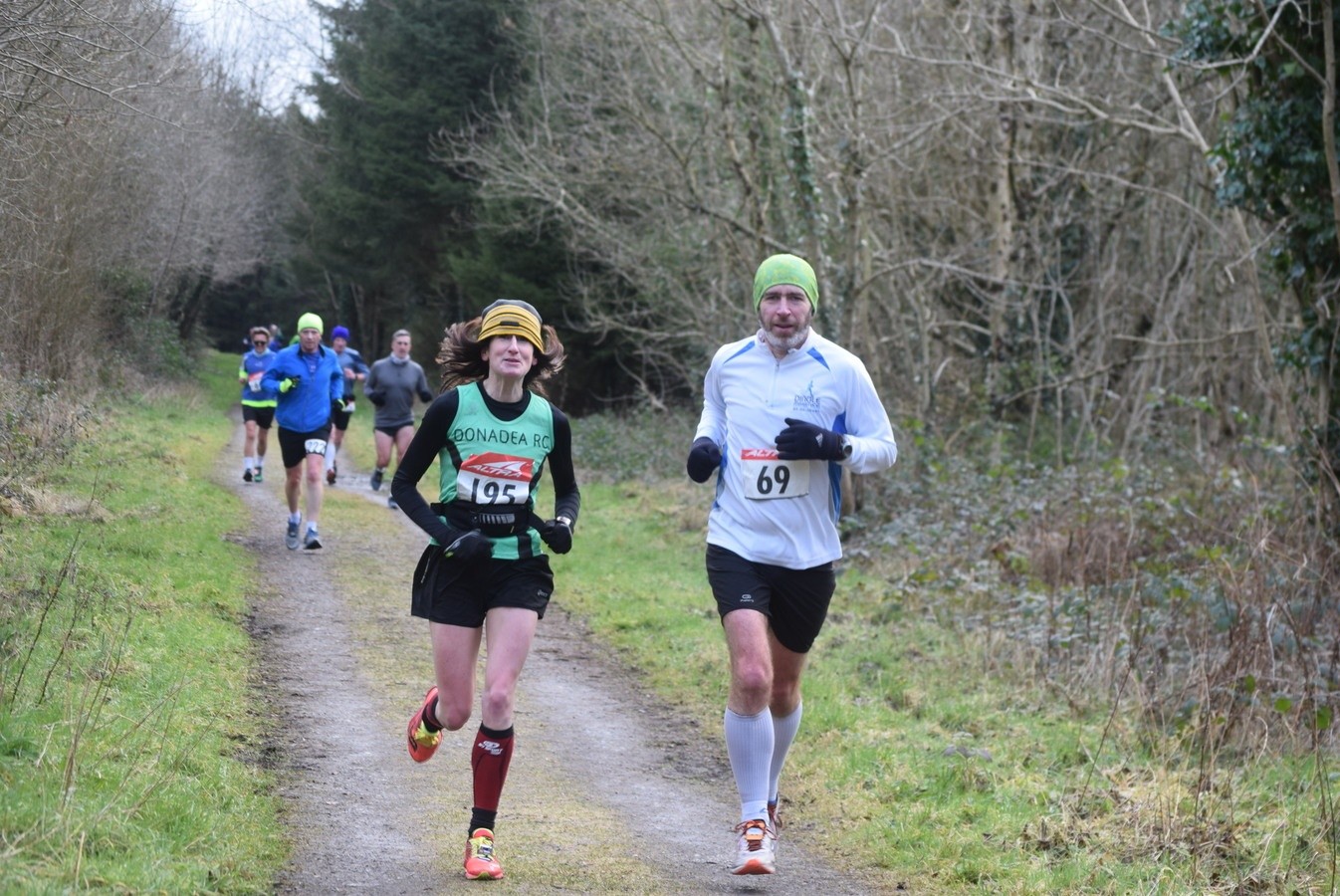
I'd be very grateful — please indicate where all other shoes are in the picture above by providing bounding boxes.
[326,460,337,484]
[371,468,385,491]
[388,496,399,509]
[243,468,252,482]
[254,466,262,482]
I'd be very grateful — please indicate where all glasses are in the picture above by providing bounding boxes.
[254,340,267,346]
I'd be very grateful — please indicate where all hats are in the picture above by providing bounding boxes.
[476,299,544,353]
[289,335,300,346]
[330,326,350,342]
[298,312,323,335]
[753,253,818,316]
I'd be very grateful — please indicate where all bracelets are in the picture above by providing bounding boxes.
[355,372,358,382]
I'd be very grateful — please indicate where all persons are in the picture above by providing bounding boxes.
[260,312,348,550]
[391,300,581,880]
[686,255,897,875]
[237,324,371,487]
[361,328,432,509]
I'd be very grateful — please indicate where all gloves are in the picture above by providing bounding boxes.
[445,532,495,568]
[419,391,432,404]
[369,392,387,406]
[333,397,349,410]
[775,418,841,461]
[686,437,722,483]
[280,375,300,393]
[544,519,573,555]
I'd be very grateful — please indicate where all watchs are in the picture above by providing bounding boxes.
[840,436,853,462]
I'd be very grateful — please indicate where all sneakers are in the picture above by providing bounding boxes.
[406,686,443,764]
[285,512,303,551]
[730,819,778,874]
[304,527,323,550]
[463,828,504,880]
[767,793,783,836]
